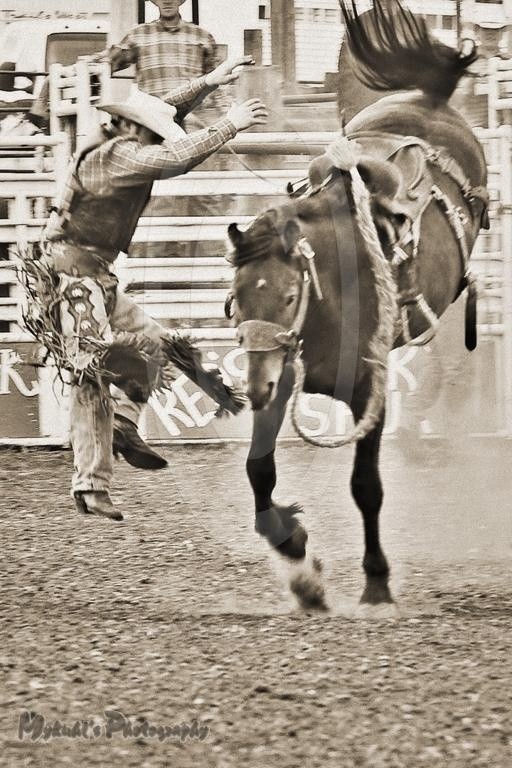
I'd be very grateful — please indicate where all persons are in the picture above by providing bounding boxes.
[35,54,268,522]
[77,0,231,329]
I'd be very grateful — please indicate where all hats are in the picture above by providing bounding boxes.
[94,90,185,142]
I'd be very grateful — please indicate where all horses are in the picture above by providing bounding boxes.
[221,1,494,619]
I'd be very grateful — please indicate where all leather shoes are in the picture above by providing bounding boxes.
[110,417,167,470]
[75,491,124,521]
